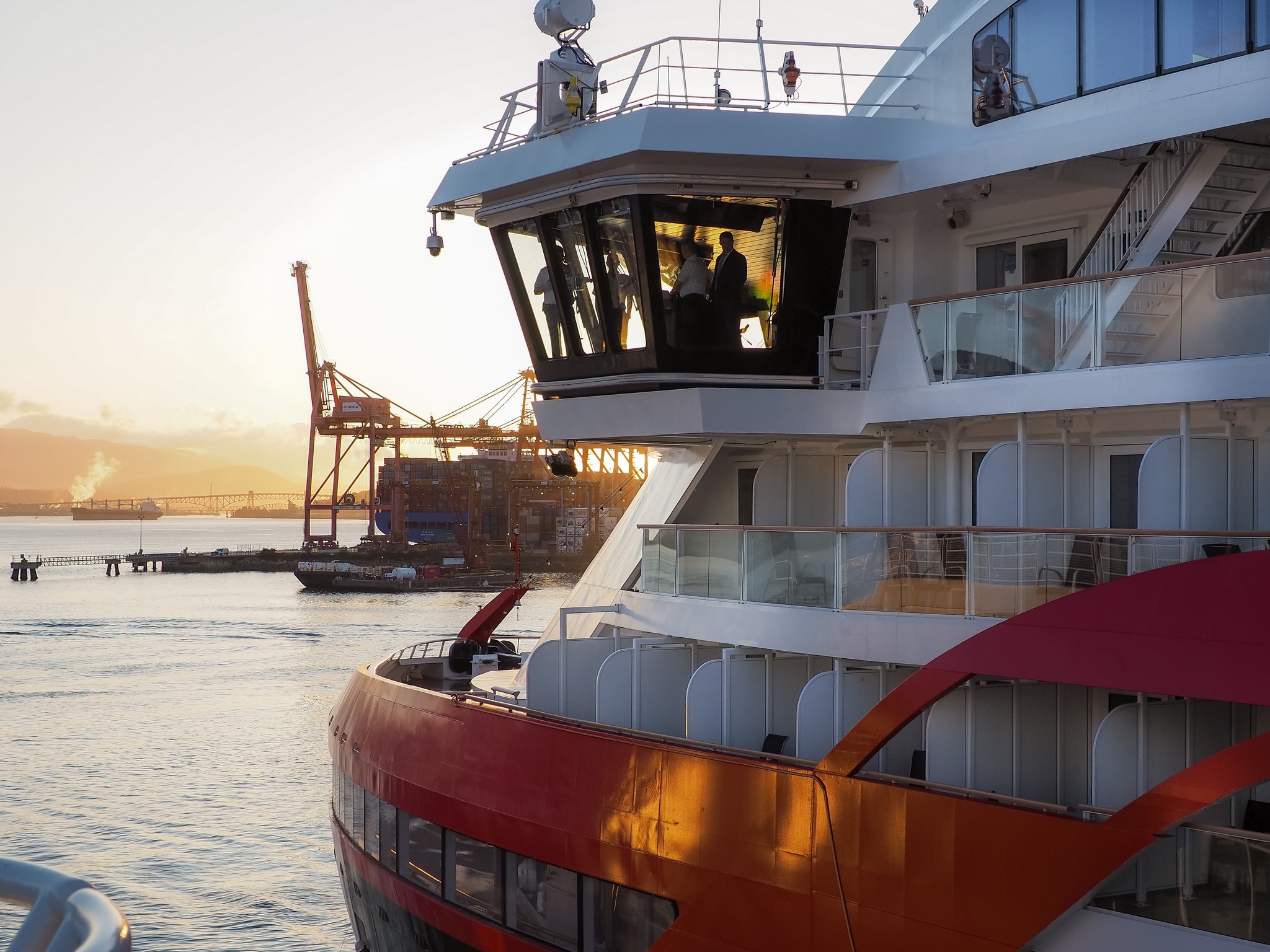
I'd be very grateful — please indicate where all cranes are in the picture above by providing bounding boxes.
[290,259,407,555]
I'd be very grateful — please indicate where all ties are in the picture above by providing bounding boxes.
[714,253,727,287]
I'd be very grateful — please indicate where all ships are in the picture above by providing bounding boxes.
[373,445,643,546]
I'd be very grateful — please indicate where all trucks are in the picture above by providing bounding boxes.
[215,549,229,556]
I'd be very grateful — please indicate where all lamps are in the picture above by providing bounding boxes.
[947,210,967,230]
[941,181,992,206]
[1119,139,1178,166]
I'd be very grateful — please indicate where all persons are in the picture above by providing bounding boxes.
[670,242,707,372]
[592,253,636,351]
[709,232,748,350]
[534,246,577,356]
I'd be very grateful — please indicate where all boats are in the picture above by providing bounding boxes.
[294,416,537,592]
[325,2,1270,951]
[70,494,162,520]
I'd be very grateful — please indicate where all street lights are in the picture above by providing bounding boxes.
[138,507,145,555]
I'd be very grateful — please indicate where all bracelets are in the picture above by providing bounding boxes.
[671,289,674,293]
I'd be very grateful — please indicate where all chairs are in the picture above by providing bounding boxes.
[760,733,790,761]
[908,749,926,787]
[887,531,969,579]
[762,528,827,606]
[1038,531,1128,587]
[1206,799,1270,904]
